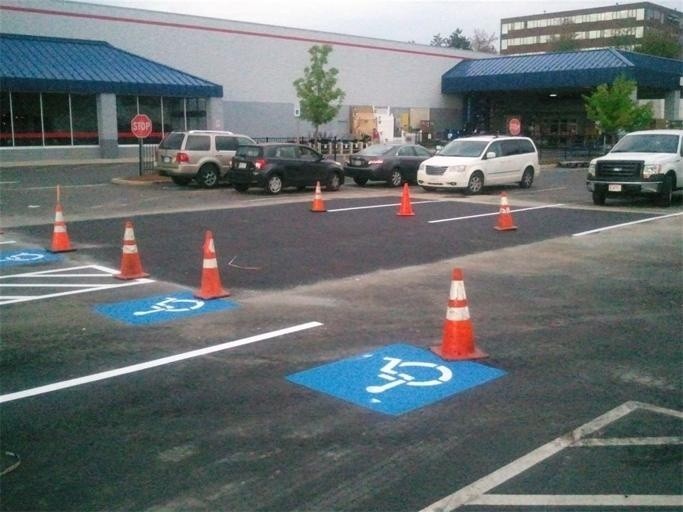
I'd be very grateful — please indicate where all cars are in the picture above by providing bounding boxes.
[230,141,345,192]
[344,143,432,187]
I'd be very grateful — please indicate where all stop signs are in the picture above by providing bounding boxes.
[510,118,521,135]
[132,113,153,138]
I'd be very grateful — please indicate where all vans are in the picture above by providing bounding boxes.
[417,136,542,194]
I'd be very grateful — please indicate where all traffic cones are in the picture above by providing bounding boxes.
[309,179,327,212]
[494,190,517,233]
[112,219,150,280]
[429,267,490,363]
[46,201,77,255]
[192,227,232,301]
[397,183,415,217]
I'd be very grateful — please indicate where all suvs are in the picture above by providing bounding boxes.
[586,129,683,208]
[154,130,258,187]
